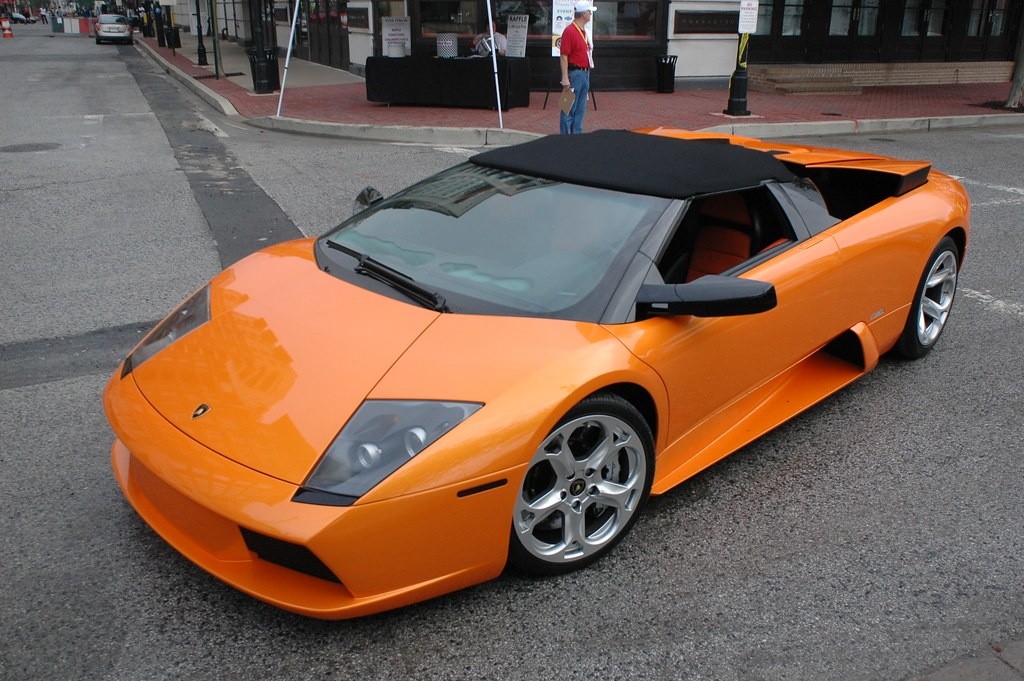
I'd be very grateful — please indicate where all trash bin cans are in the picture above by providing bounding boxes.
[656,55,678,94]
[163,27,182,49]
[245,46,281,93]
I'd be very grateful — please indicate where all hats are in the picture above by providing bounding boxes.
[574,1,597,12]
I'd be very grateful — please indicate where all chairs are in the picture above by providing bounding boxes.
[678,194,752,284]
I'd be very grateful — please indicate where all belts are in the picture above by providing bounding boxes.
[572,67,588,71]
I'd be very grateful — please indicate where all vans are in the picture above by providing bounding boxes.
[94,14,133,45]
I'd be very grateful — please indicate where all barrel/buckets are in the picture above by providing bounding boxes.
[480,36,498,54]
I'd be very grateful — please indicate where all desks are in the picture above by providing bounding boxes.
[365,54,531,113]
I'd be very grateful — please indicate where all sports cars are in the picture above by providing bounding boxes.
[99,125,973,622]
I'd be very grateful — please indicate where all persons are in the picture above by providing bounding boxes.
[23,5,33,24]
[473,21,507,57]
[79,4,95,18]
[39,5,49,24]
[52,6,63,16]
[559,0,597,134]
